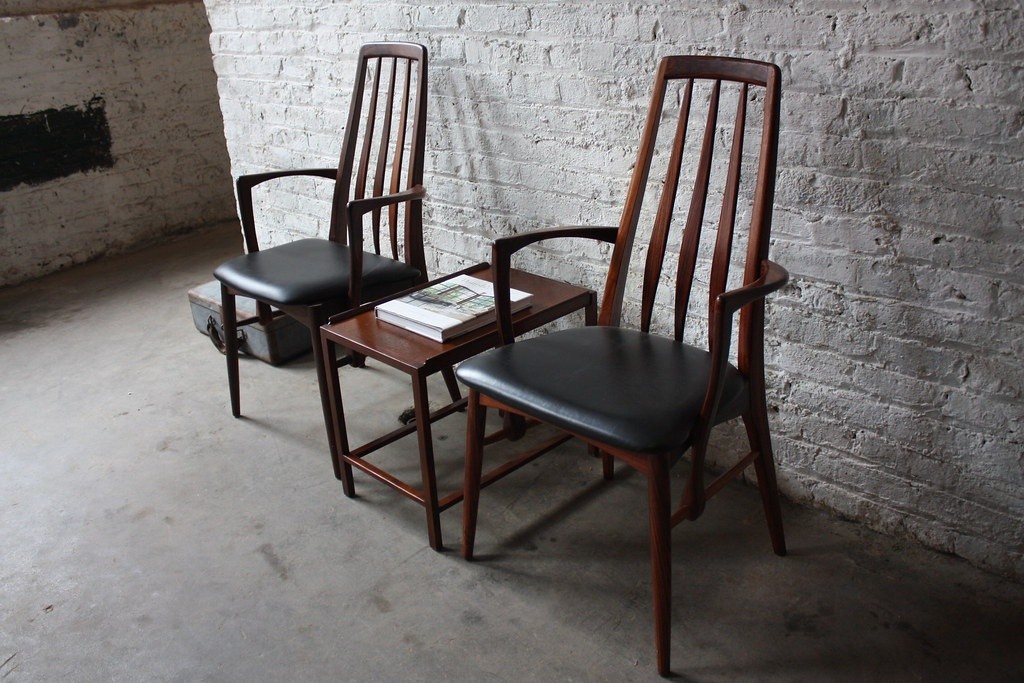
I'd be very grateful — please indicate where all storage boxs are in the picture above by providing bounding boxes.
[191,279,314,369]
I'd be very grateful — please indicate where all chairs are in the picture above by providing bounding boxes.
[457,56,791,678]
[213,40,465,480]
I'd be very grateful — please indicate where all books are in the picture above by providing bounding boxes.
[375,274,534,343]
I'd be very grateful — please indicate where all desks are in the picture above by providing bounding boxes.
[321,260,600,552]
